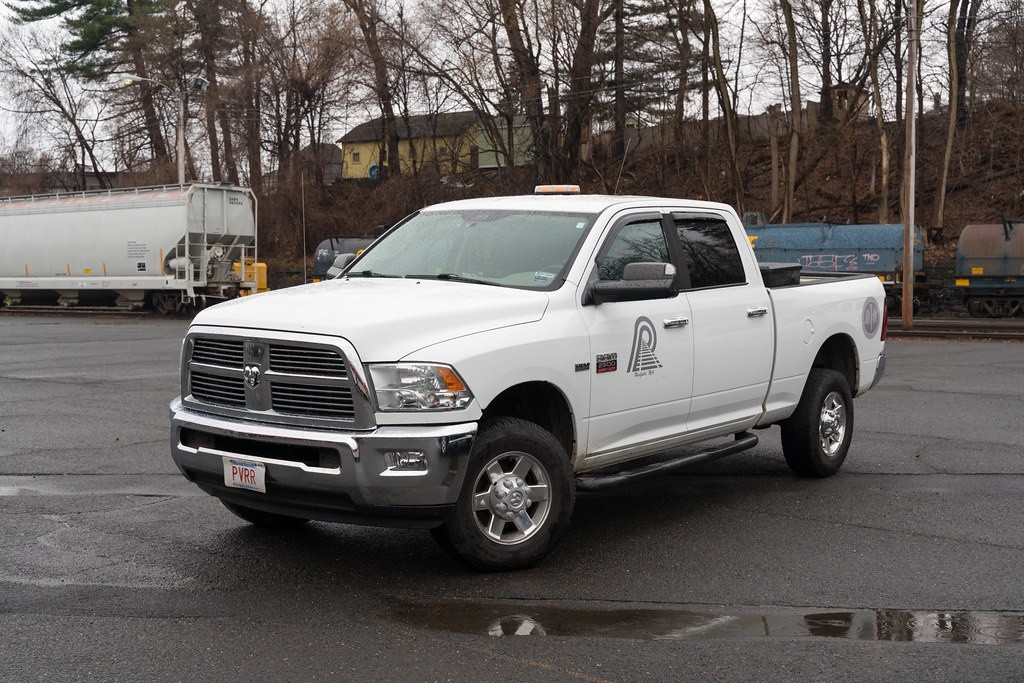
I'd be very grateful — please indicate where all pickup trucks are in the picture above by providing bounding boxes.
[169,185,888,569]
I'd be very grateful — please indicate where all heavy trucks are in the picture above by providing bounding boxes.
[0,183,259,317]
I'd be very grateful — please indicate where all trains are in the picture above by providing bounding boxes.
[310,211,1023,317]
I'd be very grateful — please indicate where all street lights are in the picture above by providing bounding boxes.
[119,72,185,182]
